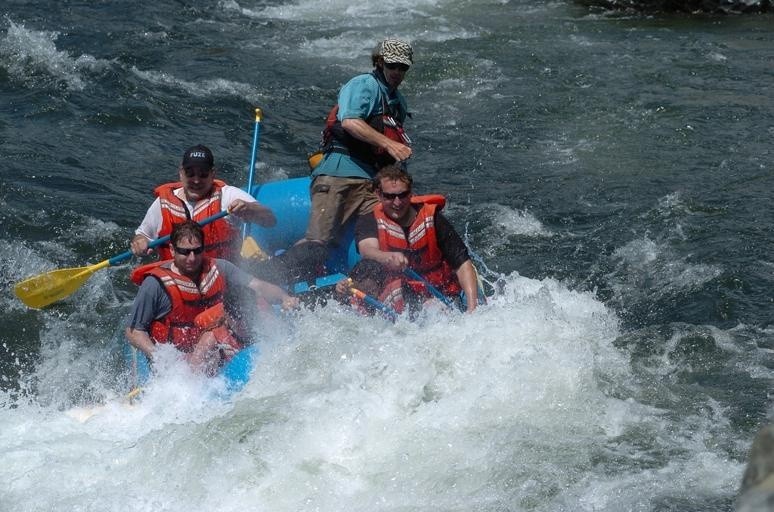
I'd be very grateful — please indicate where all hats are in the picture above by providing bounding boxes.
[182,143,215,169]
[371,38,413,67]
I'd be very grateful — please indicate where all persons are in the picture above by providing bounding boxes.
[125,219,303,384]
[128,143,277,270]
[284,258,400,325]
[249,37,415,288]
[351,165,480,320]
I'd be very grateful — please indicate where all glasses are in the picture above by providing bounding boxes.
[176,246,204,255]
[185,171,209,177]
[384,61,410,71]
[382,190,411,199]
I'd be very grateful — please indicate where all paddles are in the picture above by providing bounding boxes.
[13,206,237,310]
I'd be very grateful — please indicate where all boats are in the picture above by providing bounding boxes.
[130,176,491,398]
